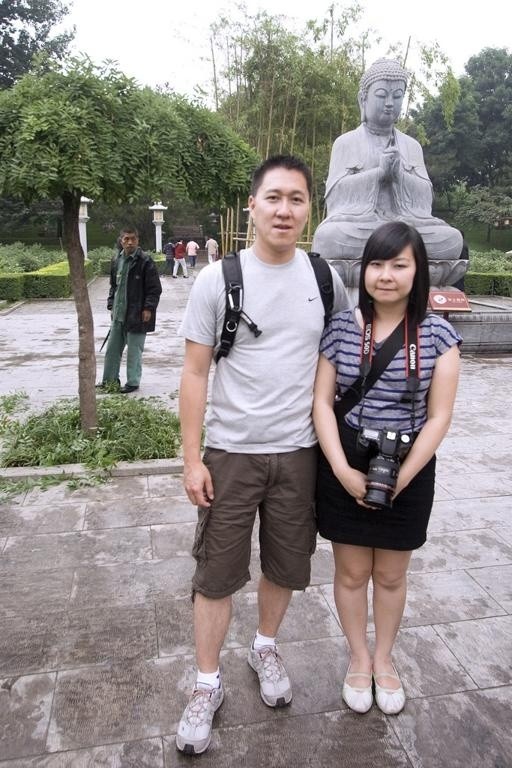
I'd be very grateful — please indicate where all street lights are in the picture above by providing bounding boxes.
[208,210,221,242]
[147,199,168,253]
[78,195,95,260]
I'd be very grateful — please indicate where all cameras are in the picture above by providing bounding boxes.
[356,427,413,510]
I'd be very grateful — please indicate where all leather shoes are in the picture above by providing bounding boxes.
[95,383,138,393]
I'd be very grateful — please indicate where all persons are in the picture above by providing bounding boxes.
[160,236,179,275]
[305,54,463,260]
[172,153,351,753]
[310,220,463,717]
[172,237,189,278]
[95,223,162,393]
[186,237,200,268]
[205,235,219,264]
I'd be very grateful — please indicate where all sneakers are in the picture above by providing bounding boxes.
[176,681,224,754]
[249,634,292,707]
[342,662,405,716]
